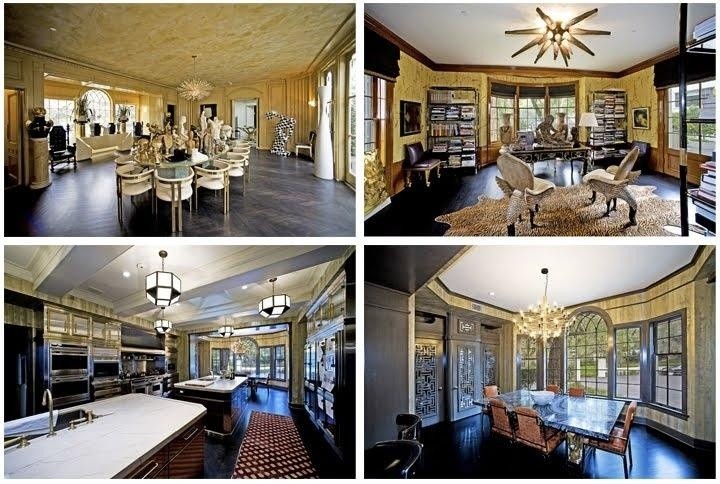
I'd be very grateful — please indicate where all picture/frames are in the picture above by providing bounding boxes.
[631,106,651,130]
[399,100,423,137]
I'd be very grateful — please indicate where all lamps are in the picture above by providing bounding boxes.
[503,4,623,67]
[577,112,600,167]
[145,250,291,339]
[511,267,576,341]
[176,55,215,104]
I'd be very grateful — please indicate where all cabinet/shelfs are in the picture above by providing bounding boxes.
[47,309,90,338]
[678,4,716,237]
[230,381,249,433]
[427,85,480,176]
[589,89,628,165]
[122,413,207,479]
[92,318,122,343]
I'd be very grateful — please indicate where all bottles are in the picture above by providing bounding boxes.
[232,369,235,379]
[229,369,232,380]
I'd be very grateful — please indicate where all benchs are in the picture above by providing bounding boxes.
[403,142,442,188]
[76,133,132,161]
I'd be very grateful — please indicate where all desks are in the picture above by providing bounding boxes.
[500,141,590,176]
[590,141,651,177]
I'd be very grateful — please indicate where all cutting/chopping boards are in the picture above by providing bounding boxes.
[185,380,214,387]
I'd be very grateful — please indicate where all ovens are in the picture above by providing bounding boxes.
[50,343,91,411]
[93,357,123,388]
[150,381,164,397]
[132,382,149,395]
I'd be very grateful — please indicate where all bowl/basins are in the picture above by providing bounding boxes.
[530,391,556,406]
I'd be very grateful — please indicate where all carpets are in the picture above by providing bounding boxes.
[226,410,318,478]
[435,182,706,237]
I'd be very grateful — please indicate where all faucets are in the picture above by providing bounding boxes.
[41,387,55,438]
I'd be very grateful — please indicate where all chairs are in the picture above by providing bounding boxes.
[364,440,424,479]
[113,138,252,233]
[294,131,315,158]
[474,384,639,479]
[582,146,643,226]
[48,125,76,172]
[495,151,554,236]
[396,414,423,441]
